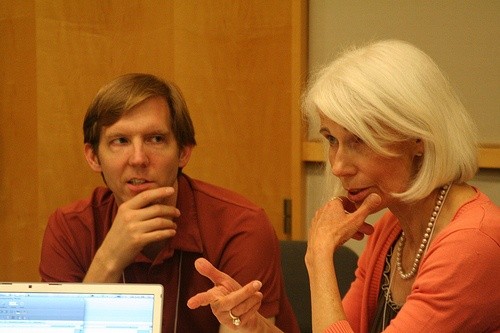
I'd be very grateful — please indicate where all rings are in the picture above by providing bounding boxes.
[228,309,241,326]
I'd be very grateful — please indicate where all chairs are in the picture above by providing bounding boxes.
[278,238,361,333]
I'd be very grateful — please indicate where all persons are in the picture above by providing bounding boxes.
[185,39,499,333]
[40,72,301,333]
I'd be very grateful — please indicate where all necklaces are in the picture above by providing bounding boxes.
[382,180,453,332]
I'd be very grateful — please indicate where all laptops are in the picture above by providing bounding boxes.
[0,281,163,333]
